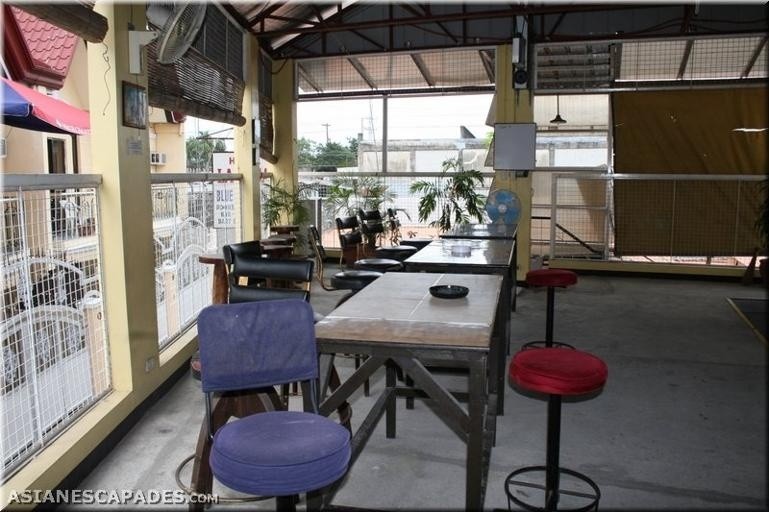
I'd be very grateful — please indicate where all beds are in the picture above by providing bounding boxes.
[313,271,500,511]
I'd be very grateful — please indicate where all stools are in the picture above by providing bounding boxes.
[504,346,609,512]
[526,269,577,351]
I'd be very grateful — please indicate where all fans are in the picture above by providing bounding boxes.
[485,189,520,225]
[128,1,206,74]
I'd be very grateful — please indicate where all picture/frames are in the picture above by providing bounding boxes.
[120,81,148,129]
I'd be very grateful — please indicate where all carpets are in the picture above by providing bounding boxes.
[725,297,768,346]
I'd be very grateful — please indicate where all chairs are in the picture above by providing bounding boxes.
[177,206,432,512]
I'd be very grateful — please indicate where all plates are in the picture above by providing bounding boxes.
[429,284,470,299]
[451,245,471,254]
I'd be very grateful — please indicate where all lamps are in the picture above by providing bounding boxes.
[550,95,567,125]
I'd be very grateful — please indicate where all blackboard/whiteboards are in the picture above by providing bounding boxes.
[493,123,536,171]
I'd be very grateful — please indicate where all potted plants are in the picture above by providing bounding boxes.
[754,178,768,296]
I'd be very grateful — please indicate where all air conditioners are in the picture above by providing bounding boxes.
[1,138,7,158]
[150,153,166,166]
